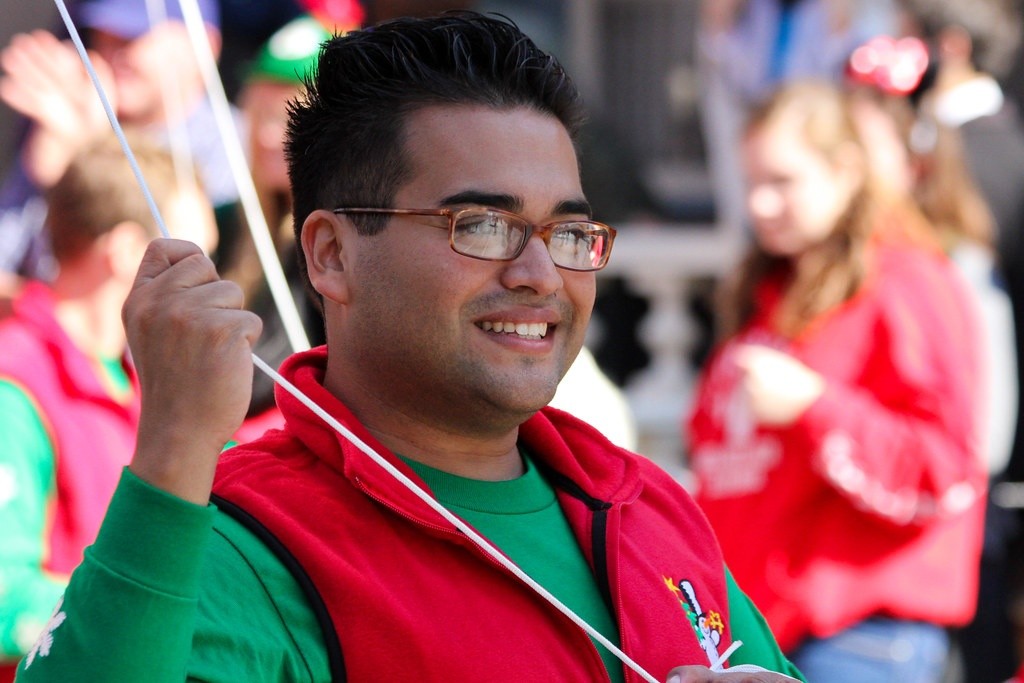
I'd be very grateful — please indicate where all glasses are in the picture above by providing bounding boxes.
[335,202,617,272]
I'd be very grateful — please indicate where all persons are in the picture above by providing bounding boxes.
[687,0,1024,683]
[0,0,369,665]
[12,8,806,683]
